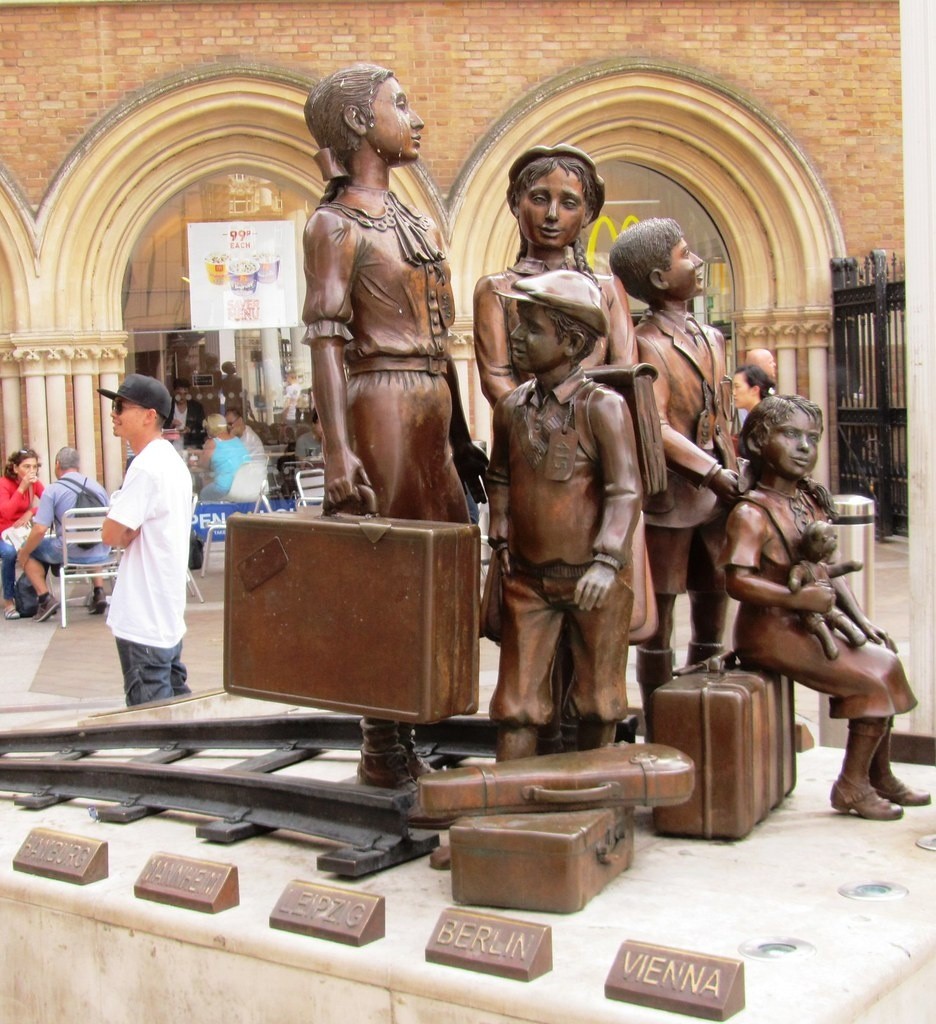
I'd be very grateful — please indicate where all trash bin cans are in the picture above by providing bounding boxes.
[795,494,876,747]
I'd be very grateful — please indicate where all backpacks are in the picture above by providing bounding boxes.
[14,572,38,617]
[54,476,105,549]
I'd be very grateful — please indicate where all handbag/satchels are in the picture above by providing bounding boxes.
[188,527,204,570]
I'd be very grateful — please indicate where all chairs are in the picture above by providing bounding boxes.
[187,494,204,602]
[49,507,125,628]
[219,452,271,503]
[202,480,273,580]
[279,461,326,510]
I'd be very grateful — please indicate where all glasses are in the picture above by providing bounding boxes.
[227,415,240,426]
[18,450,34,456]
[112,399,149,415]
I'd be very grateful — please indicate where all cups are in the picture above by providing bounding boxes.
[306,447,317,462]
[188,451,199,470]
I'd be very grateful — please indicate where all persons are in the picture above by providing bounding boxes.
[123,370,324,504]
[487,267,646,764]
[725,398,930,818]
[609,216,740,710]
[15,447,113,622]
[281,371,302,426]
[100,373,195,754]
[732,350,775,431]
[221,362,249,422]
[303,64,487,793]
[0,448,44,620]
[476,148,644,762]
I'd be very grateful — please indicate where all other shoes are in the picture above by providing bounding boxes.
[89,591,107,614]
[4,605,20,619]
[32,594,61,622]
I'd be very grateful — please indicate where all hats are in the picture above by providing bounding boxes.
[96,373,172,419]
